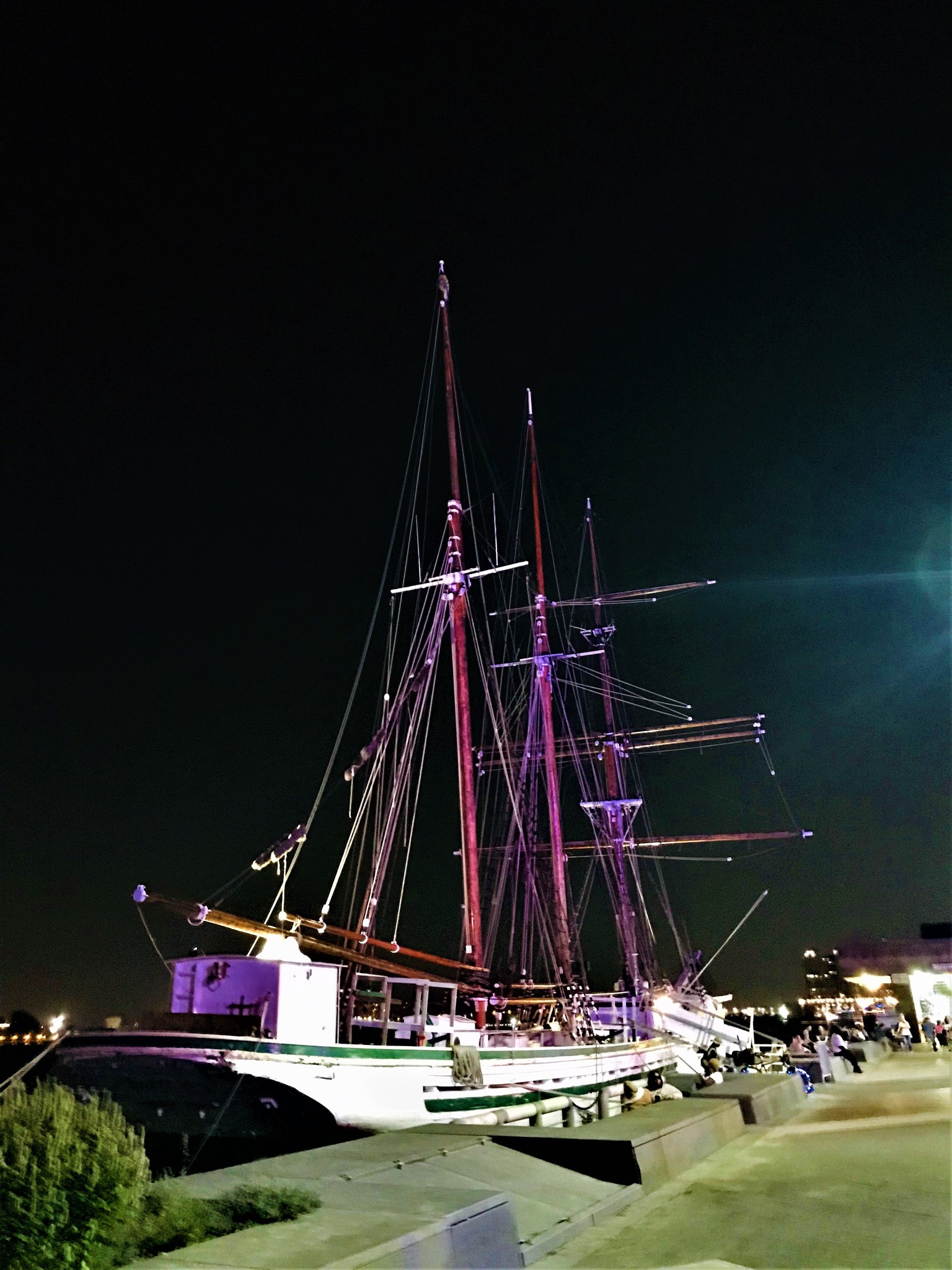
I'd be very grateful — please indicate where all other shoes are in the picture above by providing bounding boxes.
[853,1067,863,1073]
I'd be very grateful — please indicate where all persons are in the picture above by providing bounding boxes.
[788,1024,828,1055]
[833,1024,871,1042]
[875,1013,912,1052]
[831,1027,863,1073]
[644,1070,663,1092]
[921,1011,952,1051]
[701,1036,724,1086]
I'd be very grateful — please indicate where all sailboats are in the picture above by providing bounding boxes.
[54,258,814,1139]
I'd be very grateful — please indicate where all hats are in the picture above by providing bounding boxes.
[707,1058,719,1068]
[621,1081,644,1104]
[710,1037,722,1044]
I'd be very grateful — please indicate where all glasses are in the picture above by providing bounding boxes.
[656,1076,661,1080]
